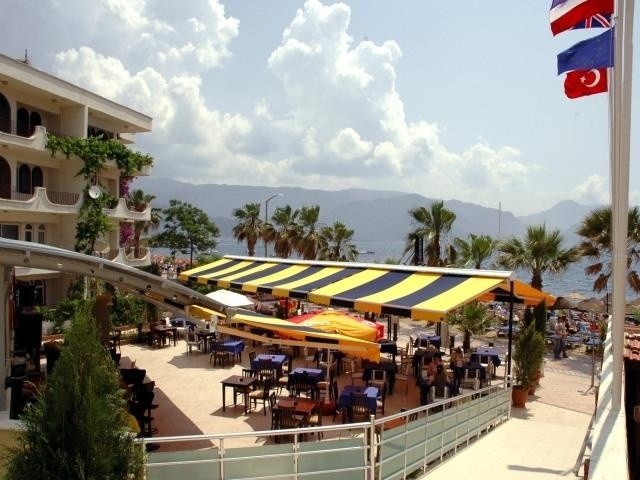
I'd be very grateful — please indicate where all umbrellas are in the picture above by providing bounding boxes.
[271,309,385,405]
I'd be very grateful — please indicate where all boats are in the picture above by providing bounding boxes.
[357,249,376,255]
[206,248,218,254]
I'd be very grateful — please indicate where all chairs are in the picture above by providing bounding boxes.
[109,329,121,365]
[210,332,501,443]
[148,320,215,353]
[497,316,603,352]
[112,368,156,453]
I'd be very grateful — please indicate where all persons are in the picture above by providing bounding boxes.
[428,353,444,376]
[427,365,452,396]
[551,308,599,361]
[254,293,263,311]
[453,346,466,396]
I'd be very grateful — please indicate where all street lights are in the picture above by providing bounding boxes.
[265,194,285,258]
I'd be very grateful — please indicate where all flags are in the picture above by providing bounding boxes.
[557,27,614,76]
[550,0,615,38]
[564,67,609,101]
